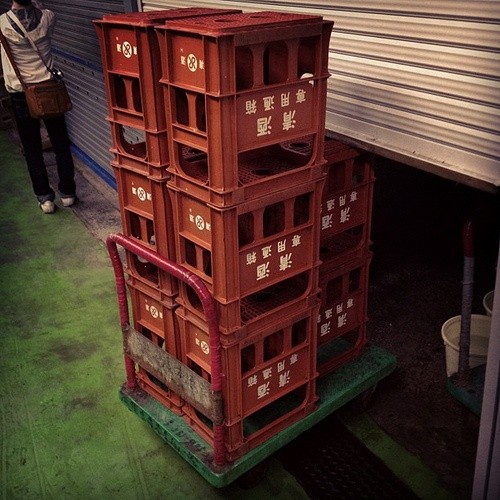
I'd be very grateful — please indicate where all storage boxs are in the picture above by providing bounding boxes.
[91,6,376,462]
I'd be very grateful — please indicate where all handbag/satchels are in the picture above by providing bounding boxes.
[23,75,74,118]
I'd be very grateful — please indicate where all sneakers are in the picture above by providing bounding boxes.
[36,200,55,214]
[60,197,74,207]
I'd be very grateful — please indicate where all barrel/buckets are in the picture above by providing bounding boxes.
[442,314,492,377]
[483,291,495,316]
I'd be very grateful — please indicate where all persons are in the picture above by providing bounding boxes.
[0,0,78,213]
